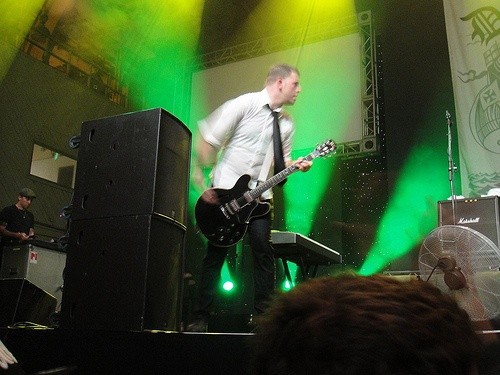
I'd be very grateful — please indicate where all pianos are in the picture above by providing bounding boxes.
[268,229,343,291]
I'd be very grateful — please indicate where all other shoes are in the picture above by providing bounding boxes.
[187,316,208,332]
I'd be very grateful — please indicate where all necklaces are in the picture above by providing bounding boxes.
[17,209,26,218]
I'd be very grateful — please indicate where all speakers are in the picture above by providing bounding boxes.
[59,107,193,332]
[0,278,58,327]
[384,194,500,321]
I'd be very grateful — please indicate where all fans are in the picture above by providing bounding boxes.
[418,224,500,321]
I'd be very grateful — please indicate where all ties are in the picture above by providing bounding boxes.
[266,104,288,187]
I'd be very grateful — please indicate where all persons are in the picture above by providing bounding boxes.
[0,188,36,241]
[186,65,312,332]
[245,274,493,375]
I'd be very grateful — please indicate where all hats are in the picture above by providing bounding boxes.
[18,187,36,199]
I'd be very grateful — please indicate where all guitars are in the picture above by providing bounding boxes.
[193,138,337,247]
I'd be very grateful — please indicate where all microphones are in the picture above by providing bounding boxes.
[446,111,452,126]
